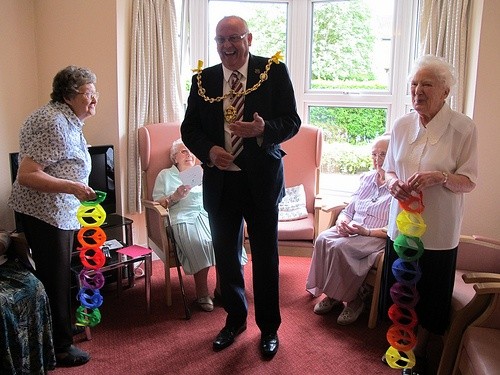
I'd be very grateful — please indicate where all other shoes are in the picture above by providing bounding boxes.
[314,297,341,314]
[196,295,214,311]
[337,303,363,325]
[56,345,91,366]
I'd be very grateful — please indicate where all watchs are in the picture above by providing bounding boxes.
[442,171,448,186]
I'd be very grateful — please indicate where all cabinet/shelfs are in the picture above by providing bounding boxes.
[70,212,152,340]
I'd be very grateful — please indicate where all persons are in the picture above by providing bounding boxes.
[170,194,177,203]
[8,65,97,366]
[180,16,301,361]
[382,54,479,375]
[305,135,392,325]
[152,139,248,312]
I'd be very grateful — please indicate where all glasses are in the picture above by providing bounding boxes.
[214,30,248,43]
[372,152,386,159]
[77,91,99,98]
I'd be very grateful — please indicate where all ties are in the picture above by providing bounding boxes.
[230,72,244,156]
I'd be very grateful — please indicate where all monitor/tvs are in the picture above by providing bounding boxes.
[10,145,116,233]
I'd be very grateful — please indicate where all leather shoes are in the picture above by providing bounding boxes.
[260,333,279,358]
[213,320,247,351]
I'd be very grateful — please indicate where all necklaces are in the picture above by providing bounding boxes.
[228,76,243,101]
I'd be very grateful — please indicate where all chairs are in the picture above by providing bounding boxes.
[138,121,202,306]
[242,123,323,258]
[321,200,398,330]
[427,234,500,375]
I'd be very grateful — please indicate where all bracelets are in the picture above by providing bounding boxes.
[386,178,392,190]
[369,228,371,237]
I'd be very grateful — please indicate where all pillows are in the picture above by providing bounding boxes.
[278,184,309,221]
